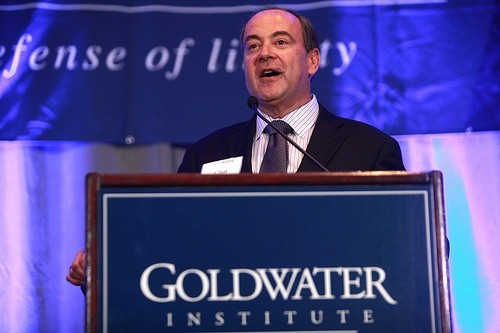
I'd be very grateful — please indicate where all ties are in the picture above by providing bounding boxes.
[259,120,294,175]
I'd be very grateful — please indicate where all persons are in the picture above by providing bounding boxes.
[67,7,451,299]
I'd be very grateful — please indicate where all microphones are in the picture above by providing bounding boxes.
[248,96,328,172]
